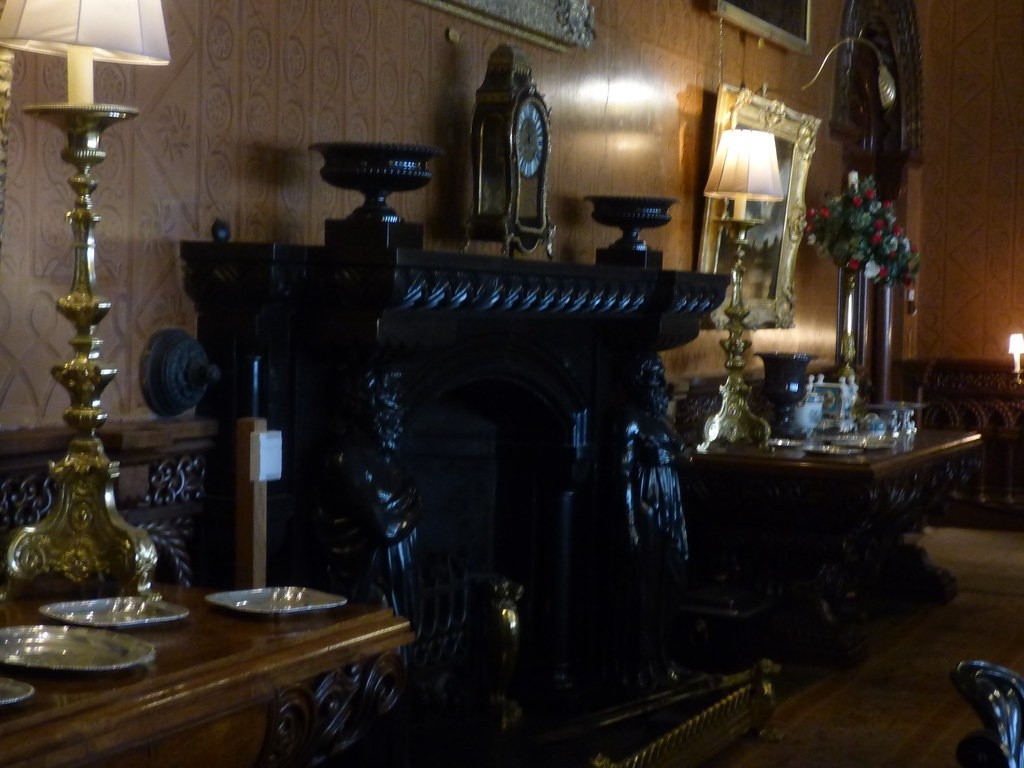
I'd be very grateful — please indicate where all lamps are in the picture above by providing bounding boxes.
[801,35,897,110]
[0,0,171,607]
[1008,333,1024,385]
[696,127,784,453]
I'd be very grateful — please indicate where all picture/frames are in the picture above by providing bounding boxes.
[709,0,814,56]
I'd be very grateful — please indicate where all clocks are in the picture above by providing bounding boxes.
[461,44,557,260]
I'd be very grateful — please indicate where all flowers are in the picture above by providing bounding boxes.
[804,172,921,317]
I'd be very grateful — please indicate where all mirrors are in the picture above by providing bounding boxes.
[696,83,822,330]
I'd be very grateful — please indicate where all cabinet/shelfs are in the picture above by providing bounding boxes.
[0,585,417,768]
[179,240,731,767]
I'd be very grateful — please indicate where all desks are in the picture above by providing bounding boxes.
[680,429,987,663]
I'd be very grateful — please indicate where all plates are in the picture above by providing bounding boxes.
[769,434,897,455]
[204,585,350,618]
[0,677,37,705]
[38,596,191,631]
[0,625,156,671]
